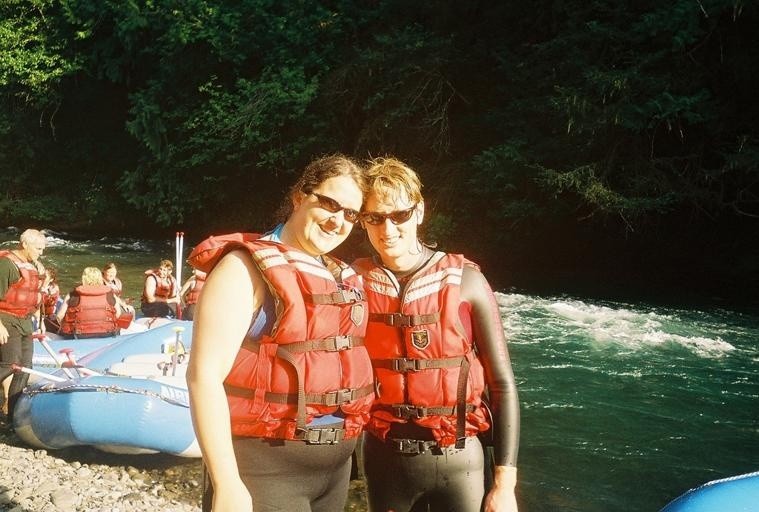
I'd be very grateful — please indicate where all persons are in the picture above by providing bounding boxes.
[184,152,375,510]
[32,260,206,340]
[352,152,522,512]
[0,228,47,421]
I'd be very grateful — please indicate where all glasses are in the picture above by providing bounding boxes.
[360,201,422,226]
[311,191,361,224]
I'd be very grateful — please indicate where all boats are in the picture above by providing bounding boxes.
[12,311,210,460]
[658,472,758,511]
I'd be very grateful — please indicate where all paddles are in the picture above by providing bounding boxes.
[116,313,133,328]
